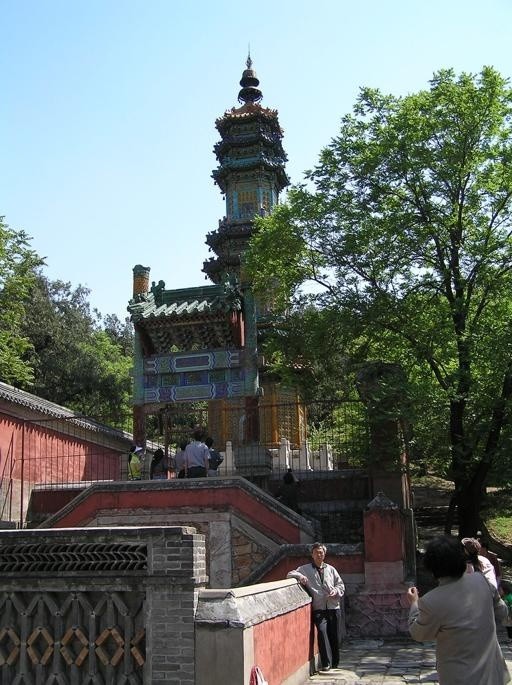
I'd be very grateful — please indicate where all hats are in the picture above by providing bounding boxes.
[462,538,481,552]
[133,446,142,453]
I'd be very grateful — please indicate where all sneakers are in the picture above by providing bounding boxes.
[322,663,337,670]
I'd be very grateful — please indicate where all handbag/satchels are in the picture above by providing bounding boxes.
[209,451,223,470]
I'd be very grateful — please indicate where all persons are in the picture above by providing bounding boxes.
[128,432,224,480]
[286,542,345,671]
[406,535,512,685]
